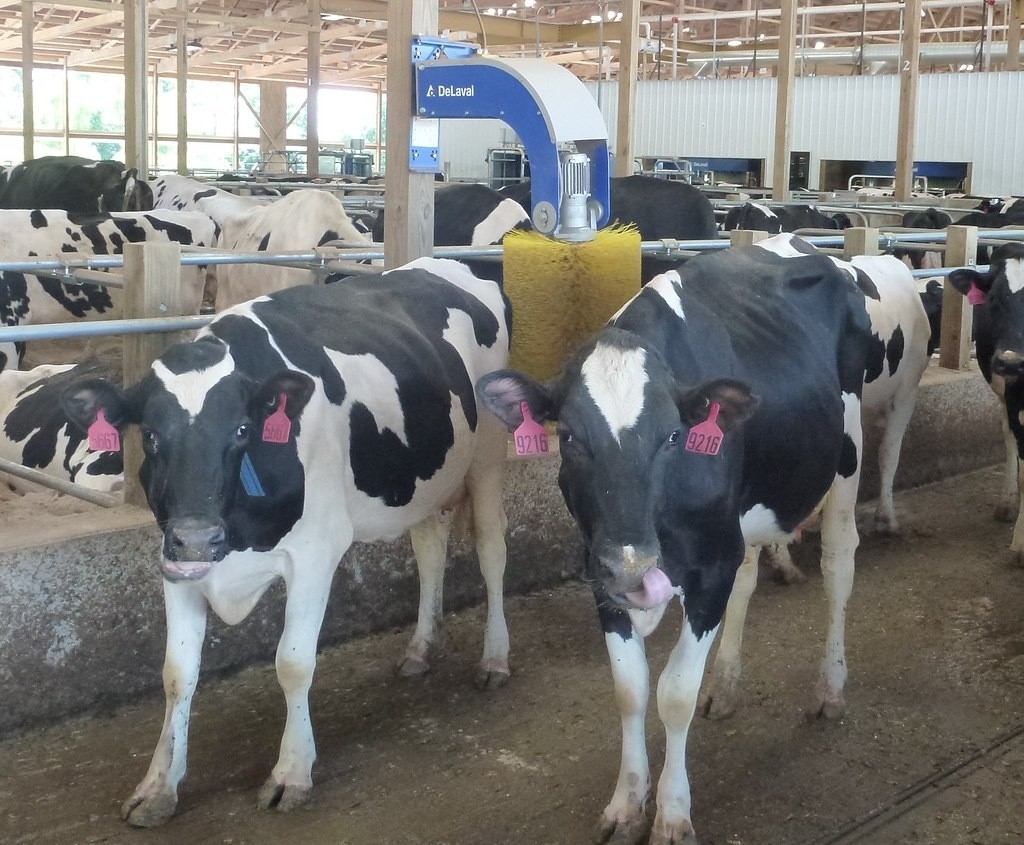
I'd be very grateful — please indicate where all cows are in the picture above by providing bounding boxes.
[2,154,1023,845]
